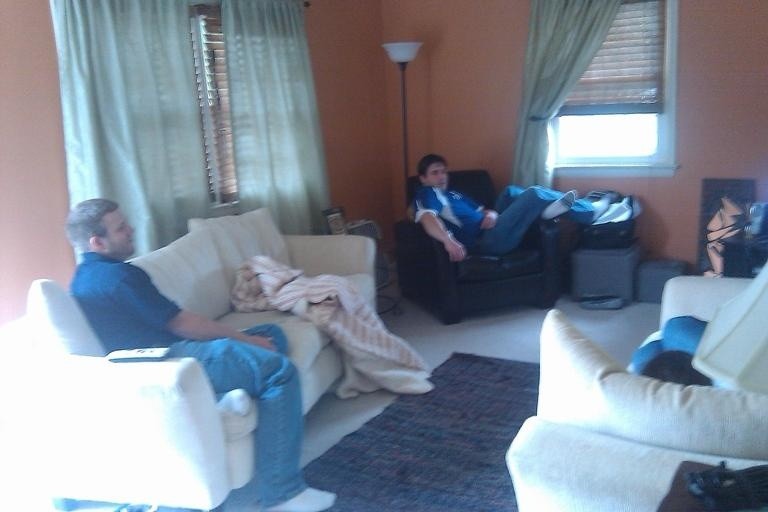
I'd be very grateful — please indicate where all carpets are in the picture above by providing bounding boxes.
[249,348,540,512]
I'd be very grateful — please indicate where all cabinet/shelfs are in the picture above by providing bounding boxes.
[697,178,755,275]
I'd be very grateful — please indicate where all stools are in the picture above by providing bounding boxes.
[568,241,690,303]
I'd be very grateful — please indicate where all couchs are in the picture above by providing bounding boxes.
[504,264,768,511]
[21,205,377,511]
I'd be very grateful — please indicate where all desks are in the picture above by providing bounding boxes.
[720,231,768,277]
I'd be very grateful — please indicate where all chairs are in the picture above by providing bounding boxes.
[398,170,554,325]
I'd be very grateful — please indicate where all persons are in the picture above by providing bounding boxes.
[632,310,732,390]
[66,198,339,512]
[411,152,621,263]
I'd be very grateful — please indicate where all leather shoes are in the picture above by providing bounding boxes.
[580,298,624,308]
[574,291,618,300]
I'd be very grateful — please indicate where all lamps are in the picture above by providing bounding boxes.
[382,42,422,204]
